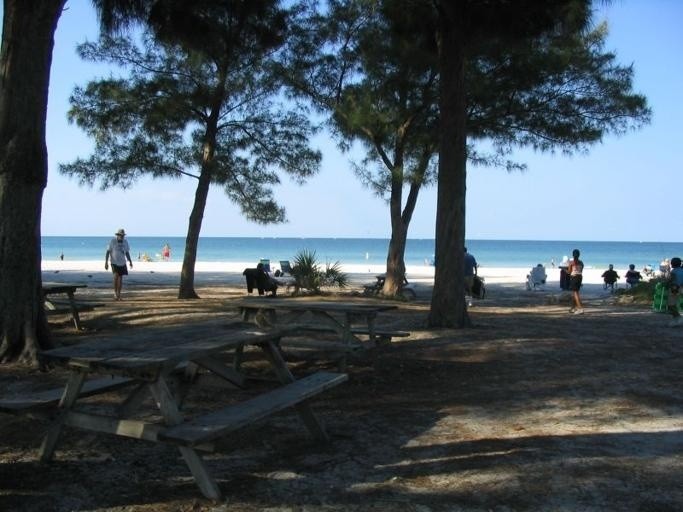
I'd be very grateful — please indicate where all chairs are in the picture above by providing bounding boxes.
[278,260,292,272]
[260,257,271,273]
[526,266,546,292]
[659,264,668,275]
[603,270,618,290]
[625,271,640,288]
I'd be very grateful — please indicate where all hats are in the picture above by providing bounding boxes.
[114,229,126,235]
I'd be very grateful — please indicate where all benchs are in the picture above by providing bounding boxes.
[0,377,138,417]
[363,274,419,298]
[158,371,351,446]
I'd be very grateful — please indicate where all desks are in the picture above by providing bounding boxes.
[38,324,331,502]
[233,298,410,382]
[41,283,104,331]
[243,269,335,297]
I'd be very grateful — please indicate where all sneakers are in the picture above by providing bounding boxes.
[574,309,584,315]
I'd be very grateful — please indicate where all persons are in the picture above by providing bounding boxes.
[161,243,171,260]
[256,263,287,297]
[526,249,682,329]
[462,248,477,308]
[103,228,133,302]
